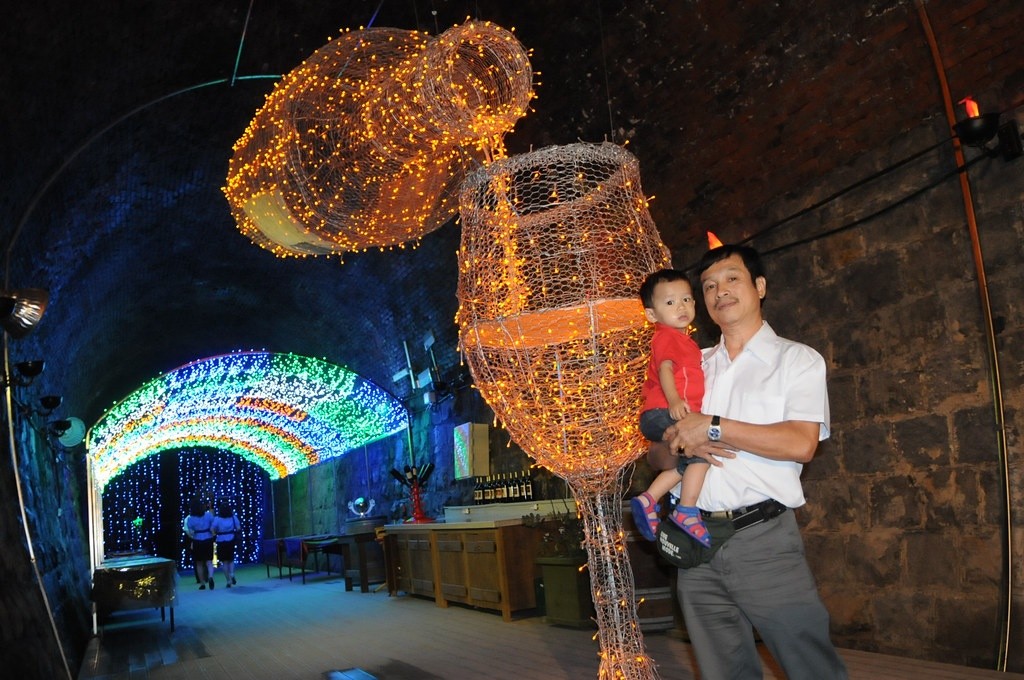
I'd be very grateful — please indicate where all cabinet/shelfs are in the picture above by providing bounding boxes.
[342,515,386,594]
[382,530,440,607]
[433,521,555,623]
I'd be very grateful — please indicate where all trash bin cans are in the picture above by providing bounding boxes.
[343,516,387,586]
[533,549,595,629]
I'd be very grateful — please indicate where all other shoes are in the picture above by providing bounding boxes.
[209,577,215,590]
[226,583,231,588]
[669,504,711,548]
[231,574,237,585]
[199,584,206,590]
[630,491,660,542]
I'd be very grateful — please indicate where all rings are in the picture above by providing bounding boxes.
[678,445,685,454]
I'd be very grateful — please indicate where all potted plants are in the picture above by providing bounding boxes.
[520,490,599,629]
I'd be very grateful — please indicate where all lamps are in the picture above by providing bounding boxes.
[31,395,64,416]
[430,382,450,397]
[0,286,49,340]
[14,360,46,387]
[49,419,71,437]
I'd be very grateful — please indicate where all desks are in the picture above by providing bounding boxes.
[92,549,182,632]
[262,537,286,580]
[284,534,345,584]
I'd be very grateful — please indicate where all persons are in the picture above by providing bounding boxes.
[630,269,712,548]
[184,500,241,591]
[646,244,850,680]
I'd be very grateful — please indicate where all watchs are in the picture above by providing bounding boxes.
[708,415,720,442]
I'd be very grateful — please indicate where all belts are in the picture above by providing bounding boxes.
[697,498,786,530]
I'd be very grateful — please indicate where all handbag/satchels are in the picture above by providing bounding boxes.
[655,509,735,570]
